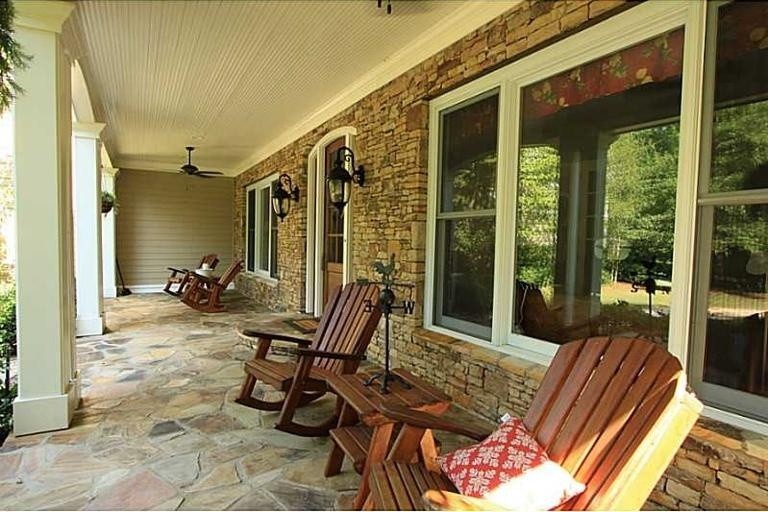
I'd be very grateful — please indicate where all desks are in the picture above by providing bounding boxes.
[324,367,453,509]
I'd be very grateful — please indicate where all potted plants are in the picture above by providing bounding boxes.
[100,190,120,217]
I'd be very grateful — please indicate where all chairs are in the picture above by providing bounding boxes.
[361,336,704,511]
[163,253,245,313]
[234,281,381,437]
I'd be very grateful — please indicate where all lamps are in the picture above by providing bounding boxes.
[271,174,300,222]
[326,146,365,218]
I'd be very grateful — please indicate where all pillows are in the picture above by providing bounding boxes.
[435,416,587,511]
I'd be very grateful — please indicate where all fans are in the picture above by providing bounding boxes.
[166,147,224,179]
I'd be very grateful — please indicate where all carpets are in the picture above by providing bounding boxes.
[282,317,321,334]
